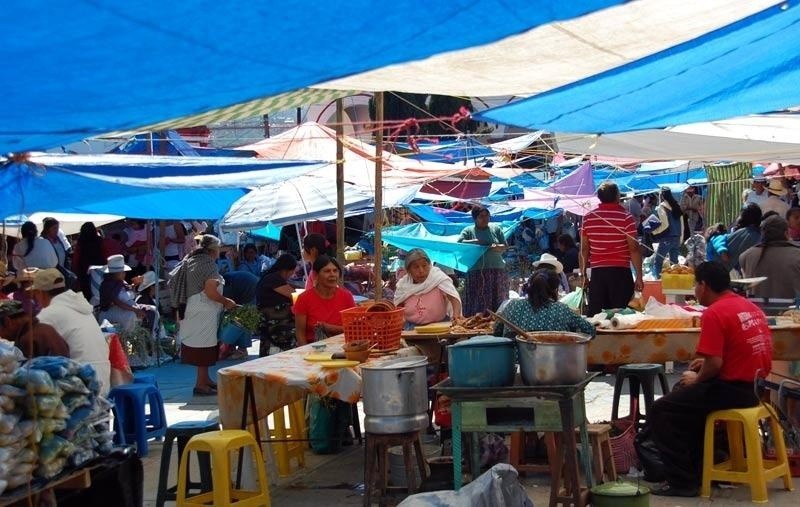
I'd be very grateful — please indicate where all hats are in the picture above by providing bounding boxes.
[102,254,166,292]
[532,253,564,275]
[749,175,788,196]
[0,261,66,319]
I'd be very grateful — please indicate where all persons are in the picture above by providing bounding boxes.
[629,186,703,278]
[706,176,799,315]
[26,268,112,398]
[581,182,644,318]
[647,260,773,496]
[515,213,581,293]
[395,248,462,331]
[495,268,596,453]
[178,235,297,394]
[457,205,510,317]
[13,217,165,338]
[257,234,404,446]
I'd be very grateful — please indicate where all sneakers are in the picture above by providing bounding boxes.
[649,480,699,497]
[226,351,248,360]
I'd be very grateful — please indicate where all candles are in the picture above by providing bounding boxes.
[291,291,298,306]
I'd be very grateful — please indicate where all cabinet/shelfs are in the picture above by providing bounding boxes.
[0,277,40,337]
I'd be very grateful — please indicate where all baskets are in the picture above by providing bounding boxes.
[601,399,642,474]
[340,306,407,353]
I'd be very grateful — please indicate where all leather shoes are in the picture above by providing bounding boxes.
[196,381,217,395]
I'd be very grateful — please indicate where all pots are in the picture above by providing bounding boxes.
[515,330,595,387]
[358,355,432,435]
[588,452,651,507]
[445,330,514,390]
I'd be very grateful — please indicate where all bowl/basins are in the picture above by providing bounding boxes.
[342,340,371,351]
[365,300,397,330]
[425,456,464,478]
[345,350,370,362]
[312,344,327,352]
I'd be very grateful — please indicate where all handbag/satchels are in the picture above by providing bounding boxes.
[579,305,590,315]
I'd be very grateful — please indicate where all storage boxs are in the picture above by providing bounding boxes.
[642,281,666,305]
[659,274,694,289]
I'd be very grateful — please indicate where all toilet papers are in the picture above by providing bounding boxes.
[611,314,646,329]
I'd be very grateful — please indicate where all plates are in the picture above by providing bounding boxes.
[414,326,450,334]
[304,354,331,362]
[321,360,359,367]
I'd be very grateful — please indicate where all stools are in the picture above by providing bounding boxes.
[611,363,669,433]
[107,383,167,458]
[269,398,309,449]
[133,373,160,404]
[549,424,617,507]
[364,430,430,507]
[510,431,556,477]
[701,401,795,502]
[176,429,271,507]
[247,402,304,477]
[156,420,221,507]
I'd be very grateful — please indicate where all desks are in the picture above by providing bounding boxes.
[1,444,143,506]
[345,257,375,292]
[662,277,770,304]
[429,371,603,506]
